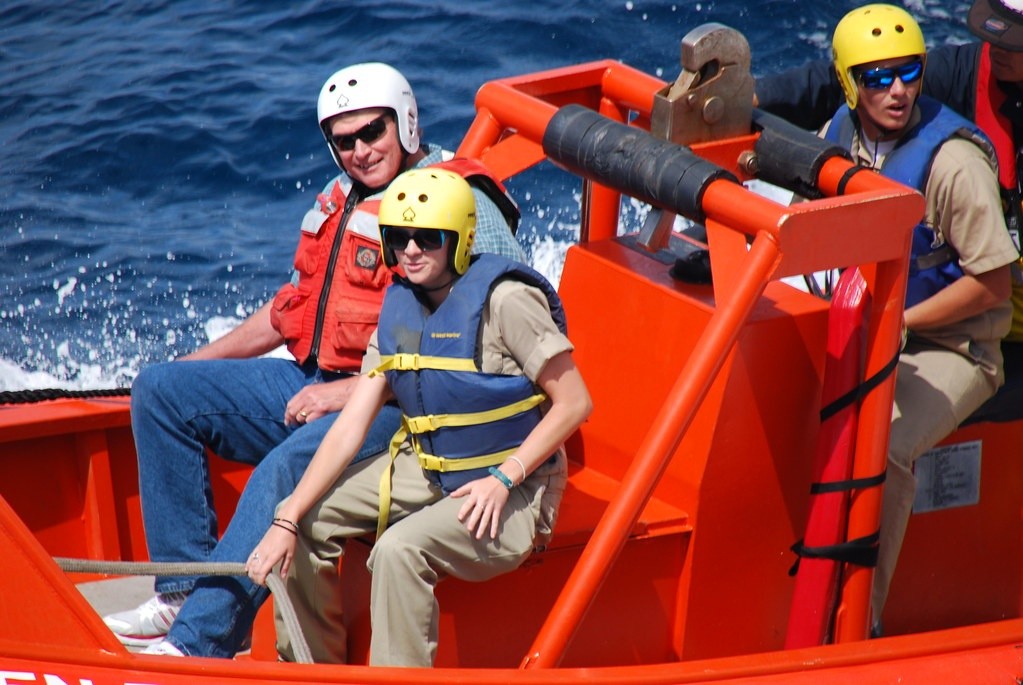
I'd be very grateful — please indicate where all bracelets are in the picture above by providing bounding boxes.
[489,467,514,494]
[271,523,297,536]
[273,518,298,530]
[507,456,526,481]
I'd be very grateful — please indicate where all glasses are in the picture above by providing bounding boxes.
[328,112,388,151]
[384,228,451,251]
[852,60,921,89]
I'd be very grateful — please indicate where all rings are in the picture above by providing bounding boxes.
[253,553,260,561]
[299,410,308,418]
[477,503,484,510]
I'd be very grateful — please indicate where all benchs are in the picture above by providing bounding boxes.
[209,425,693,670]
[884,341,1023,636]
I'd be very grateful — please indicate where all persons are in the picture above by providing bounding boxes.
[752,0,1023,646]
[242,166,593,667]
[102,61,527,659]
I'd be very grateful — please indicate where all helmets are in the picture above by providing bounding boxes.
[377,167,476,276]
[317,62,419,171]
[831,4,926,110]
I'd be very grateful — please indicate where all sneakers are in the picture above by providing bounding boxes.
[138,642,183,656]
[101,592,194,646]
